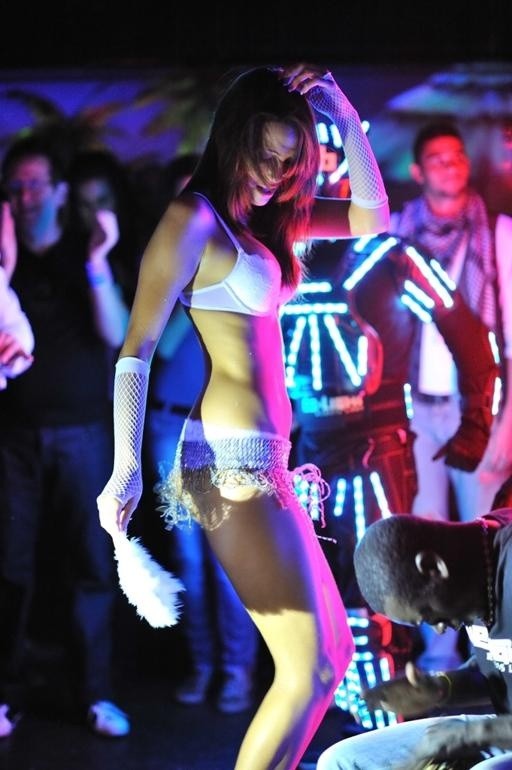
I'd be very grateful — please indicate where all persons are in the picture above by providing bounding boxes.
[384,119,511,673]
[0,135,260,737]
[96,60,391,770]
[318,506,512,770]
[278,117,502,730]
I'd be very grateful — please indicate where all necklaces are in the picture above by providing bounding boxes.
[475,513,497,630]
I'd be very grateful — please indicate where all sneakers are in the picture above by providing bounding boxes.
[216,668,252,715]
[88,698,131,738]
[175,666,212,706]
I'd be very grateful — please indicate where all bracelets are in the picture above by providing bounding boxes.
[438,669,455,706]
